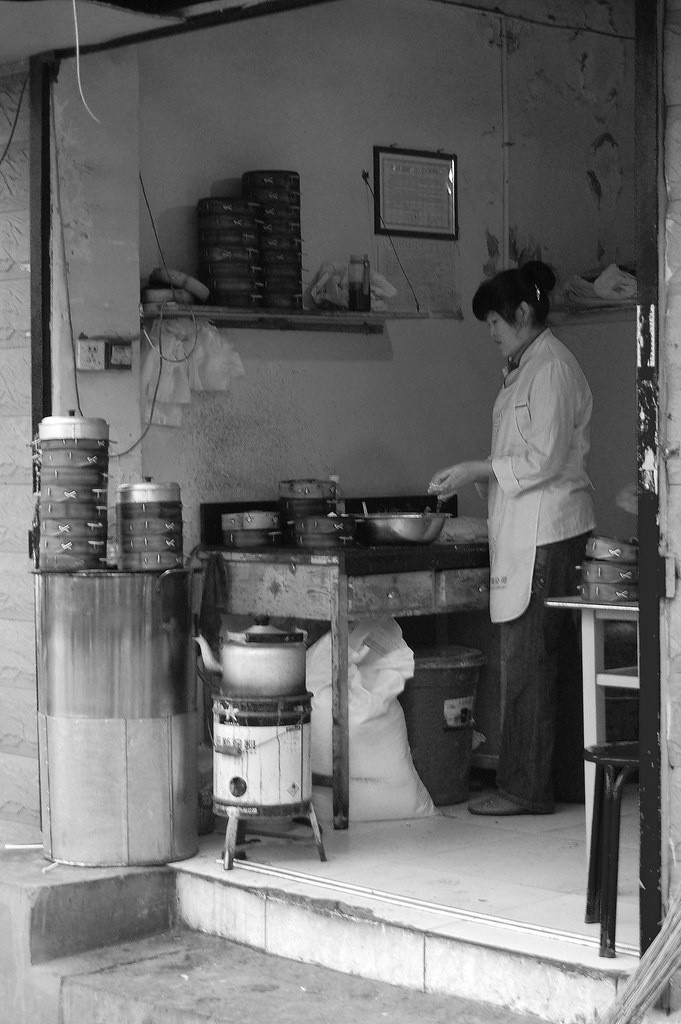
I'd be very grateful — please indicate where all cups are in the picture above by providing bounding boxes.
[348,254,372,311]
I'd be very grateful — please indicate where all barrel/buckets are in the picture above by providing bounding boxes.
[387,644,488,804]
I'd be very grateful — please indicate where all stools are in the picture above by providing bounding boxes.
[576,741,643,958]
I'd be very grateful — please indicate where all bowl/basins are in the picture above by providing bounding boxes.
[346,513,451,546]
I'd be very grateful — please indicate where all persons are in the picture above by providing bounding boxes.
[427,262,595,815]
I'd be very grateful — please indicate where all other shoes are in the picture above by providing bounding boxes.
[553,785,583,802]
[468,796,537,815]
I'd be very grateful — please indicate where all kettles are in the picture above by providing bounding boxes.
[190,613,308,695]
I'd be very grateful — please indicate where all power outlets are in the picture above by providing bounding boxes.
[75,338,105,370]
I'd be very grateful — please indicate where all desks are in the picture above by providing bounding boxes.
[196,542,493,833]
[542,592,639,871]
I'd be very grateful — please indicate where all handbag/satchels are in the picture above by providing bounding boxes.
[311,263,397,311]
